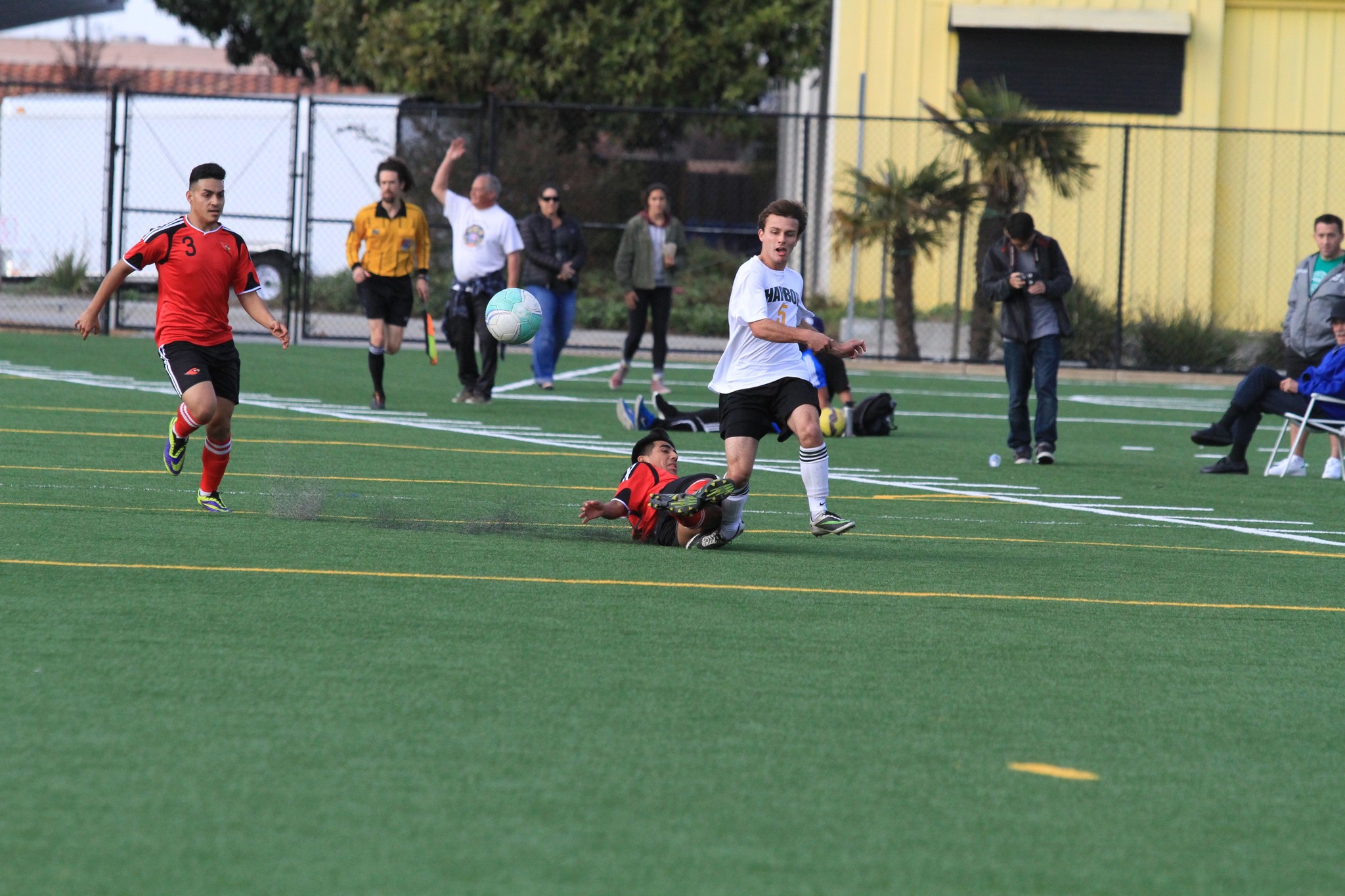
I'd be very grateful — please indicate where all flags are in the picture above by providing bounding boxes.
[422,309,438,365]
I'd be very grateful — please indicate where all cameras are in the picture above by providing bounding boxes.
[1017,271,1038,288]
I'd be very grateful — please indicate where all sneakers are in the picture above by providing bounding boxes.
[617,397,637,431]
[697,520,745,550]
[1268,458,1308,476]
[682,478,736,517]
[371,392,385,410]
[163,416,189,476]
[652,379,671,394]
[648,492,701,515]
[196,486,231,513]
[635,395,655,431]
[1191,423,1234,446]
[1322,457,1342,479]
[609,365,628,389]
[809,509,857,538]
[652,391,676,421]
[1201,456,1250,472]
[1014,446,1034,464]
[1036,442,1056,464]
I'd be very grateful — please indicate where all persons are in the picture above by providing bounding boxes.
[616,315,856,431]
[345,137,683,414]
[578,430,735,548]
[74,161,291,515]
[979,212,1075,467]
[1192,214,1345,479]
[684,199,866,551]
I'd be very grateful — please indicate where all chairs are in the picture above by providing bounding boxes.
[1264,392,1345,479]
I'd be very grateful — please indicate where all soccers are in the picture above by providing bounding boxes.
[818,407,846,439]
[483,287,542,344]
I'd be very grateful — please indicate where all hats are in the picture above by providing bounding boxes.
[1326,301,1345,323]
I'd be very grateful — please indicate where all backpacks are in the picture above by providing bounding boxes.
[851,393,898,436]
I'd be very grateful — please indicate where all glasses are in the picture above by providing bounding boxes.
[541,196,560,202]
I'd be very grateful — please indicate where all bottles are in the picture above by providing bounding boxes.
[844,402,856,437]
[989,454,1002,467]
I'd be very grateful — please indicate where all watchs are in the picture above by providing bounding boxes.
[418,275,430,282]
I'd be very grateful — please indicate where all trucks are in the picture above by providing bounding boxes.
[0,88,478,315]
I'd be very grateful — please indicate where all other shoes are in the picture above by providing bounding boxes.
[539,381,554,389]
[464,390,492,405]
[451,387,473,403]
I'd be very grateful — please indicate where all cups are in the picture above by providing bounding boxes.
[663,243,677,259]
